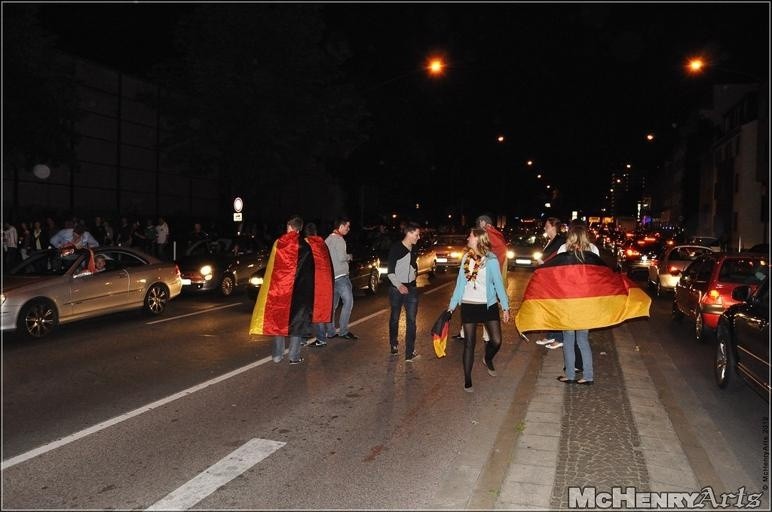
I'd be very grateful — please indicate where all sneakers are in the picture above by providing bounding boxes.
[577,379,594,385]
[308,340,328,348]
[556,375,576,383]
[391,344,398,355]
[406,350,420,362]
[463,382,473,392]
[482,356,496,377]
[289,357,305,365]
[273,354,284,363]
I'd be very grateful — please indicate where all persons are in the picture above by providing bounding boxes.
[323,220,359,341]
[536,217,565,351]
[532,220,618,384]
[558,240,600,373]
[451,215,507,343]
[447,228,509,393]
[387,222,423,362]
[304,224,327,348]
[188,224,207,244]
[272,217,315,365]
[232,245,239,255]
[4,216,170,274]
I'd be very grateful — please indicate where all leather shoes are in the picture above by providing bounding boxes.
[536,339,555,345]
[327,334,338,339]
[452,333,463,340]
[545,342,563,350]
[339,332,358,339]
